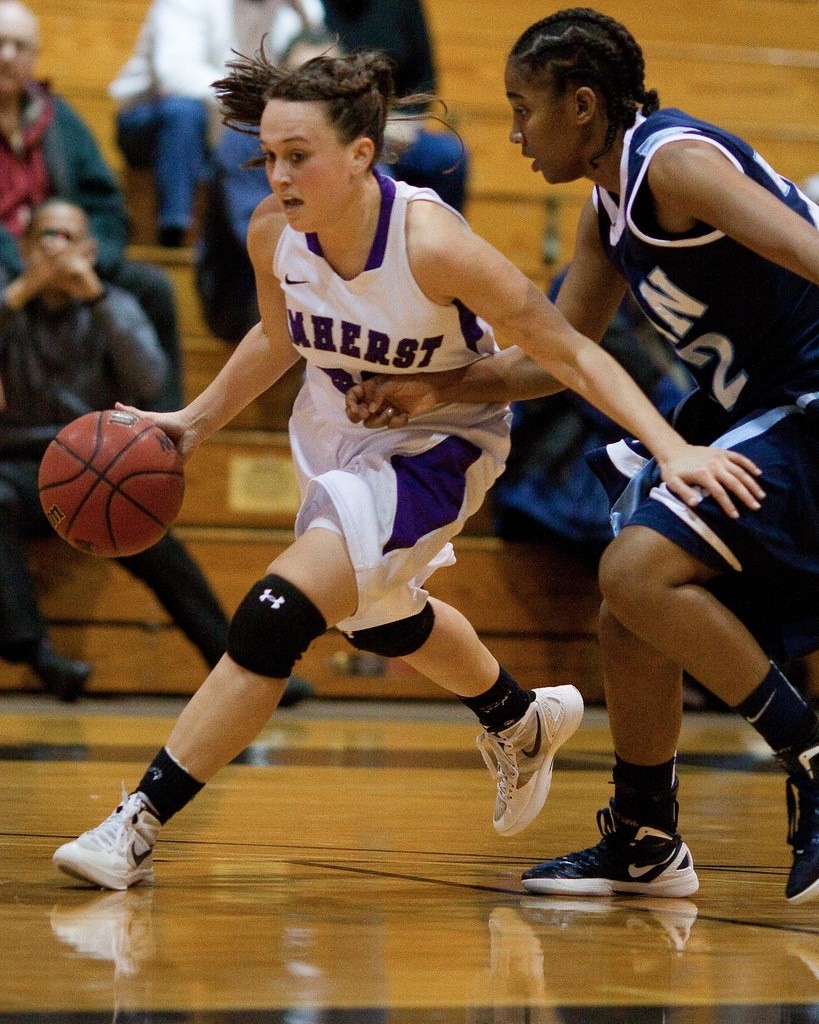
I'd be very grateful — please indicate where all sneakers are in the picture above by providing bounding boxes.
[518,900,698,958]
[475,682,583,837]
[520,802,698,901]
[53,784,164,888]
[51,883,153,959]
[774,726,819,905]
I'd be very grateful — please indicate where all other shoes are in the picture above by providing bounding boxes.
[279,678,310,707]
[48,662,90,699]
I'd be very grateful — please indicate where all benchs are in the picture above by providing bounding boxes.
[0,0,819,706]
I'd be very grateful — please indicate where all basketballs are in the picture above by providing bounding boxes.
[37,408,187,561]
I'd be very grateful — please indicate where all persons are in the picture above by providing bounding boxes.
[340,10,819,905]
[51,35,768,898]
[0,1,819,699]
